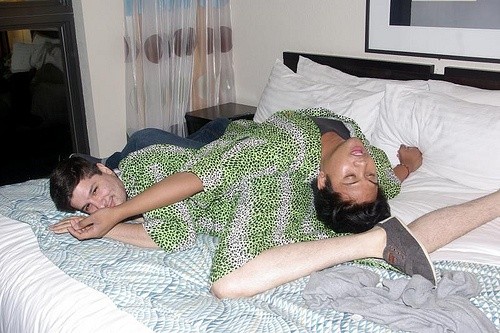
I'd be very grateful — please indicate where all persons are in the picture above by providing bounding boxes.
[67,106,424,241]
[48,128,500,300]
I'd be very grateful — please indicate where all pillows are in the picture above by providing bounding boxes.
[253,57,384,142]
[371,84,499,187]
[298,55,427,102]
[428,79,499,108]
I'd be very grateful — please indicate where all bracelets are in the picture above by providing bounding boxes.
[396,163,410,178]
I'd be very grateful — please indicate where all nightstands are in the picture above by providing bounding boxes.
[185,102,257,137]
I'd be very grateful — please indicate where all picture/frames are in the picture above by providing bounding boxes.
[365,0,500,61]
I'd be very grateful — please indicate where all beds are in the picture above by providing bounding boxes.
[0,51,500,333]
